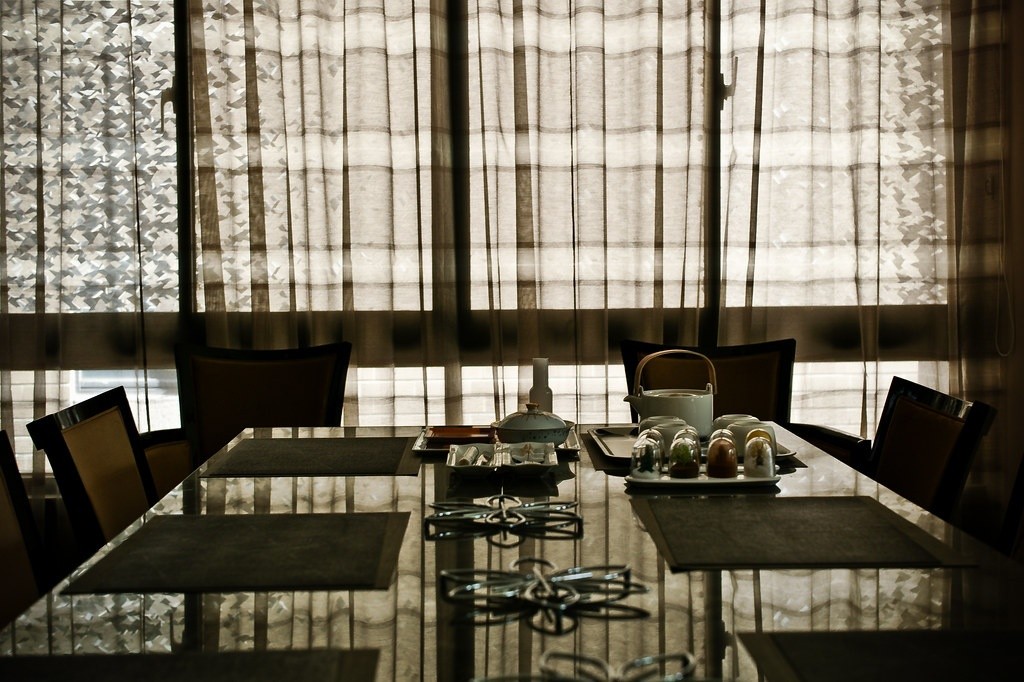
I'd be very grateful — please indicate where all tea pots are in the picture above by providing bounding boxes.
[623,349,717,444]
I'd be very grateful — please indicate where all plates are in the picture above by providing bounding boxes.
[411,427,581,454]
[624,473,781,483]
[587,427,796,458]
[423,428,494,445]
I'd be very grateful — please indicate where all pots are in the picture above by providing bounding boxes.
[490,404,575,448]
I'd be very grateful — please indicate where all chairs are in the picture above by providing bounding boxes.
[0,340,1024,623]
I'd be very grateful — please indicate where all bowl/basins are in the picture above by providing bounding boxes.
[446,442,559,480]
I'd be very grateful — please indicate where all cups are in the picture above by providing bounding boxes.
[706,414,776,478]
[630,416,701,479]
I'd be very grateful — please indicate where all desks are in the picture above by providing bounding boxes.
[0,422,1024,682]
